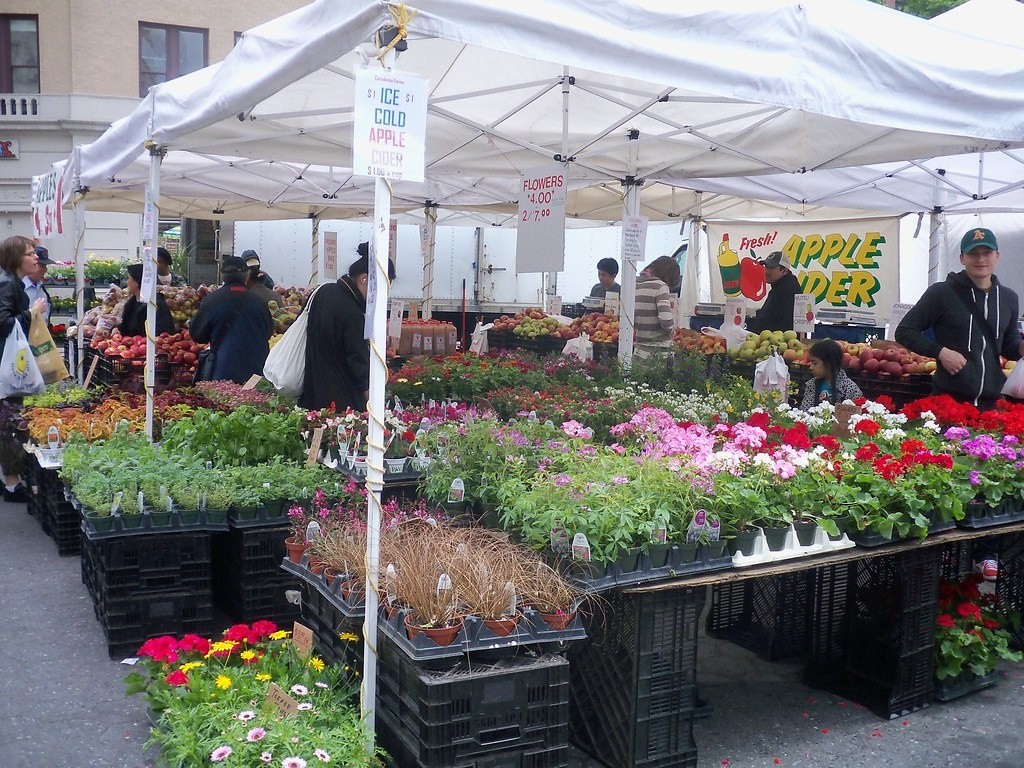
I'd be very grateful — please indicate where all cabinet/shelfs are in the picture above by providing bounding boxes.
[41,272,118,350]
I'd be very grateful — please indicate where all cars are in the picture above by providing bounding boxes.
[44,278,96,338]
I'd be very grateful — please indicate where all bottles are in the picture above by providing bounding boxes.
[385,316,457,357]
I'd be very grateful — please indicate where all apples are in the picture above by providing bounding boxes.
[66,284,312,376]
[674,329,727,354]
[490,308,620,343]
[727,330,1016,379]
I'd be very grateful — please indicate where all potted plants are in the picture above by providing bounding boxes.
[0,387,613,680]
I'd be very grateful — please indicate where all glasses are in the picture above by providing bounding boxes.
[24,251,36,256]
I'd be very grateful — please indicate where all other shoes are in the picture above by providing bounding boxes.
[3,483,28,502]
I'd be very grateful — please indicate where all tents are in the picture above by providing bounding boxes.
[54,0,1024,768]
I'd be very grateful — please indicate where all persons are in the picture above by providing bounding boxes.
[799,339,863,409]
[242,249,274,289]
[1,234,58,502]
[190,255,281,386]
[157,247,186,288]
[633,255,680,360]
[298,242,397,413]
[581,257,621,320]
[746,250,808,339]
[894,229,1024,581]
[101,264,177,337]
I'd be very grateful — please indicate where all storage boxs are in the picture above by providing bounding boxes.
[27,304,1024,768]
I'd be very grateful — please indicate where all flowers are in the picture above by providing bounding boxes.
[42,254,1024,768]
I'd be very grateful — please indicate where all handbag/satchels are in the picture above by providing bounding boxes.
[262,308,306,397]
[28,305,69,385]
[193,350,214,385]
[0,317,46,399]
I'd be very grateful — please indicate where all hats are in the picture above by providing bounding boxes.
[221,256,247,272]
[759,251,791,270]
[245,258,260,266]
[34,246,56,264]
[961,228,998,254]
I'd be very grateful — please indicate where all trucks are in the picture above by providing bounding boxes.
[178,215,690,355]
